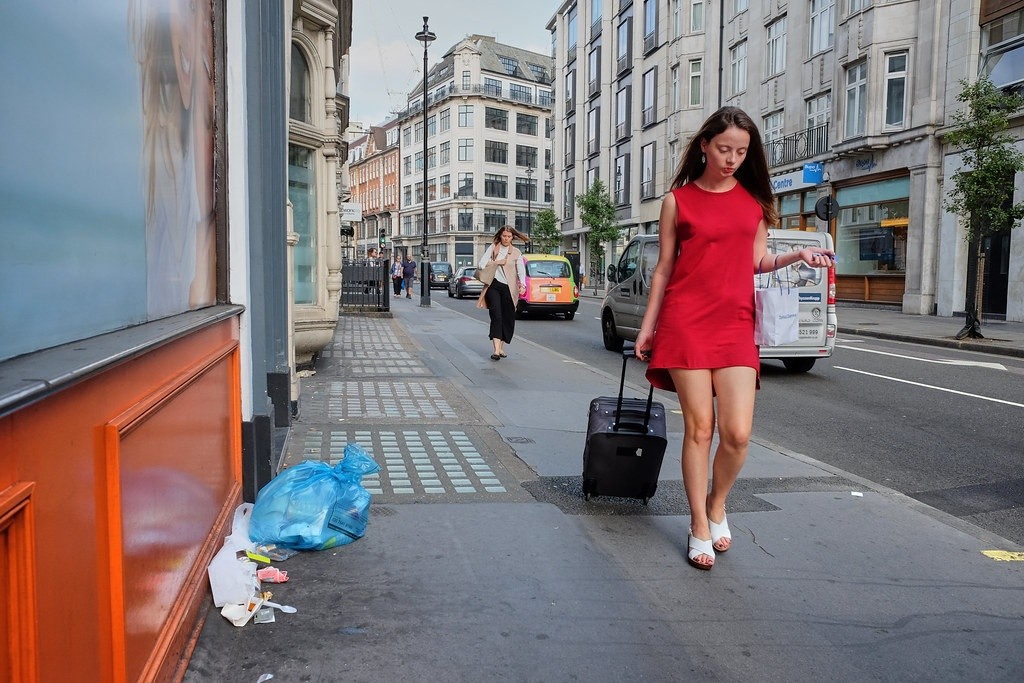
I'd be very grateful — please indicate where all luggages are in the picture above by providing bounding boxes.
[582,350,667,506]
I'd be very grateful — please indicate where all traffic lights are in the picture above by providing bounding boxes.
[378,228,388,249]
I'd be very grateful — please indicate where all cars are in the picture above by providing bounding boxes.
[517,254,580,319]
[447,266,485,299]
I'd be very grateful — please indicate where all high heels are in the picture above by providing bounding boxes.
[687,520,715,571]
[707,504,732,553]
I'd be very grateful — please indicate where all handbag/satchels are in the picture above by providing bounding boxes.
[582,275,586,283]
[392,274,397,278]
[401,279,405,290]
[473,243,498,287]
[754,254,799,346]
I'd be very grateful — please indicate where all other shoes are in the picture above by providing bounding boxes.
[406,293,411,299]
[394,294,401,298]
[490,354,500,361]
[499,352,507,358]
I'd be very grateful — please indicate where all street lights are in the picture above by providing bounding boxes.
[414,15,439,309]
[525,162,534,254]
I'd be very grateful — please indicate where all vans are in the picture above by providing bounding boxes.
[429,262,453,290]
[601,229,837,374]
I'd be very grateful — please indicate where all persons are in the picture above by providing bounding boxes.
[635,106,836,571]
[390,255,402,298]
[364,248,380,294]
[402,255,417,299]
[376,252,384,294]
[476,225,530,360]
